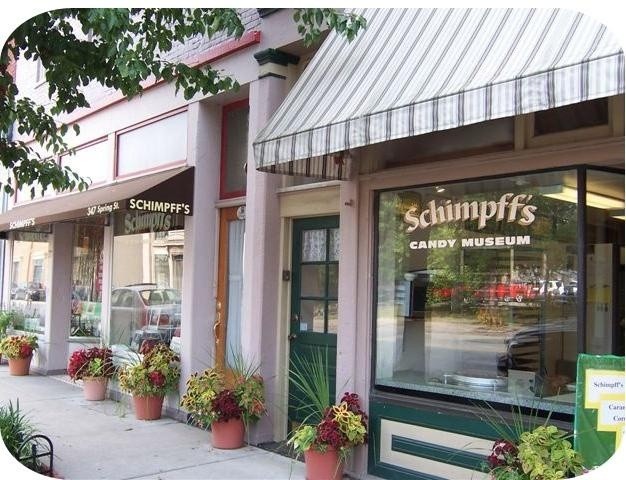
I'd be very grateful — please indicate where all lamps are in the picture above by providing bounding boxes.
[435,185,445,192]
[609,210,625,219]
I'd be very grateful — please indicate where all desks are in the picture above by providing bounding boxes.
[539,185,625,210]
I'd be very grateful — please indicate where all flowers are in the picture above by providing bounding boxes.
[446,381,591,480]
[178,340,271,434]
[67,347,115,382]
[117,340,180,399]
[0,335,39,359]
[275,341,370,480]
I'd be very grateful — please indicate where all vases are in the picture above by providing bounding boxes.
[133,391,163,420]
[83,377,106,401]
[8,358,27,375]
[211,414,245,449]
[304,444,343,480]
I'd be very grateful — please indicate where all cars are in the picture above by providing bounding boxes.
[11,282,182,349]
[435,276,578,304]
[498,321,577,383]
